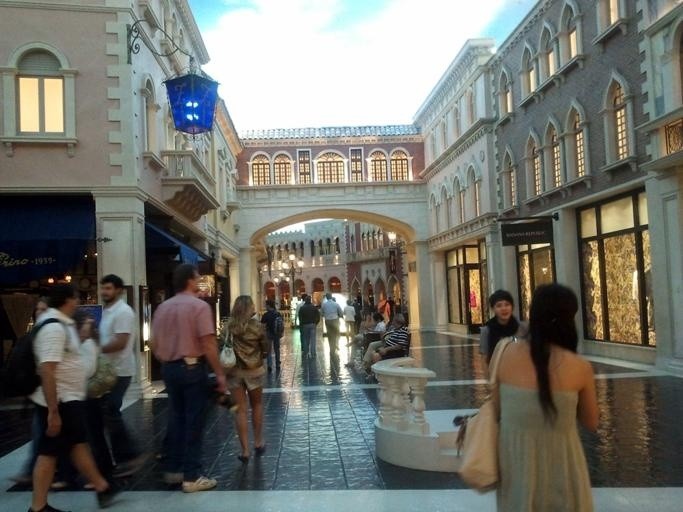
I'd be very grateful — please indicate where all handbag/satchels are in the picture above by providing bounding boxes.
[217,326,238,370]
[453,335,517,491]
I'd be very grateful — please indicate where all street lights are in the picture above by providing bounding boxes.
[162,57,222,140]
[283,254,303,297]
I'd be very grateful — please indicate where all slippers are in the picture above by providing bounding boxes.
[238,451,251,464]
[254,440,267,451]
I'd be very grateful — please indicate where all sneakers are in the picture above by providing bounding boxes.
[183,476,217,493]
[96,477,129,508]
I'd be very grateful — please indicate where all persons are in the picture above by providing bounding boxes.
[487,284,603,512]
[479,289,531,375]
[85,273,136,466]
[25,281,129,511]
[149,263,228,492]
[219,295,271,463]
[261,292,409,373]
